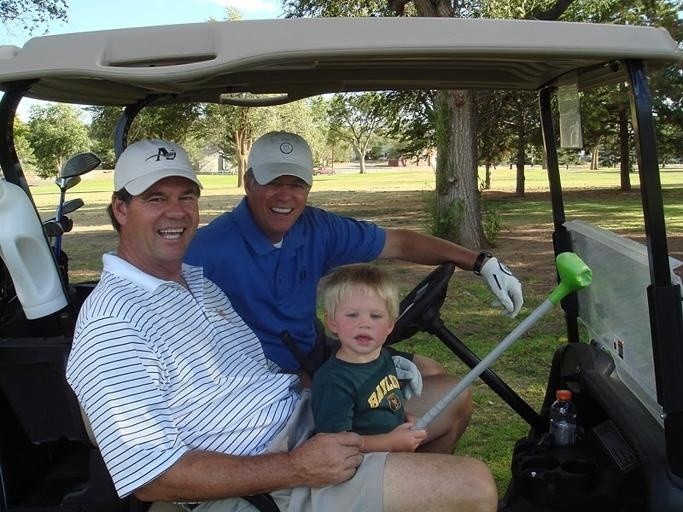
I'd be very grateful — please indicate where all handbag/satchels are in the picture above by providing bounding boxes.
[510,428,597,505]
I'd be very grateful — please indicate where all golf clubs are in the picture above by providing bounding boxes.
[41,153,101,264]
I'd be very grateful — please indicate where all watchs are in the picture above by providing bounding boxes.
[473,250,493,276]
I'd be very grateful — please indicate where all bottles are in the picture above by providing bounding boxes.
[549,389,579,449]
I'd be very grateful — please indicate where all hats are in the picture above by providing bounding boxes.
[246,130,313,188]
[113,138,203,197]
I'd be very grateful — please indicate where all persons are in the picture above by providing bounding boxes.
[183,131,524,393]
[311,264,428,451]
[65,139,498,512]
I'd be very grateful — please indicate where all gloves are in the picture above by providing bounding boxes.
[480,257,524,320]
[391,355,423,401]
